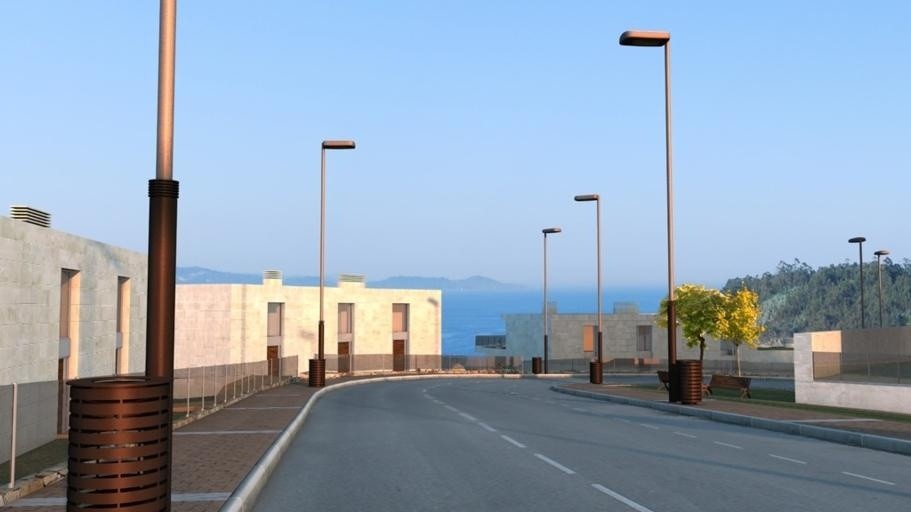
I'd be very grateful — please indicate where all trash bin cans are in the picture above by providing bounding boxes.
[67,378,173,512]
[532,357,542,374]
[590,362,602,383]
[677,359,702,404]
[309,359,326,387]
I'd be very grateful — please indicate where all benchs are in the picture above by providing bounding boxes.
[658,370,713,400]
[710,373,754,400]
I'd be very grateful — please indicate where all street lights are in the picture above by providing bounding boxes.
[874,250,890,328]
[575,195,602,362]
[849,238,865,328]
[543,228,561,374]
[620,31,677,403]
[319,140,355,360]
